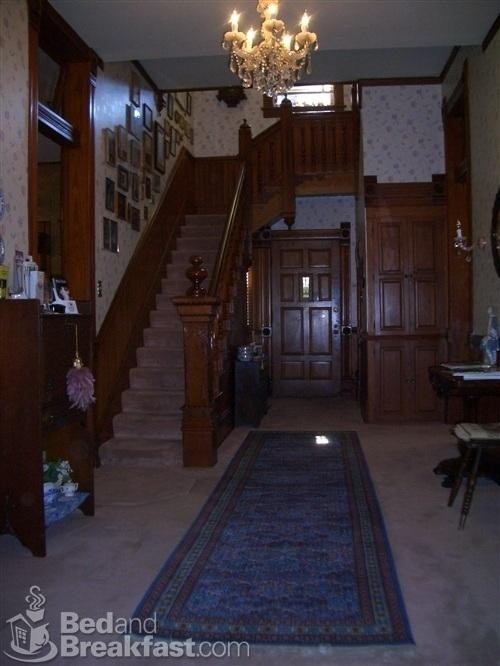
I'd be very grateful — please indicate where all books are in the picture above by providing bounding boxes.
[463,374,500,382]
[440,361,491,370]
[443,369,500,376]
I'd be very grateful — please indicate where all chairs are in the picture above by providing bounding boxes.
[446,420,500,529]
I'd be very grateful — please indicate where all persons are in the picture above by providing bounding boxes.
[56,283,75,301]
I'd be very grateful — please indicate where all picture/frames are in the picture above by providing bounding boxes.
[101,70,194,253]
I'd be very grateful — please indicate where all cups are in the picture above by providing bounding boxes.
[59,481,79,497]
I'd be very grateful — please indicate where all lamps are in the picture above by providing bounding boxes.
[452,219,488,265]
[220,0,320,98]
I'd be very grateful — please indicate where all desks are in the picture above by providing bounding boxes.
[235,355,269,428]
[429,363,500,489]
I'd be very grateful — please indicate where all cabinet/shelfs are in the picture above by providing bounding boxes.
[0,300,96,558]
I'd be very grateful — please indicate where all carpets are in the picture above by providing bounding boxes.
[122,430,416,646]
